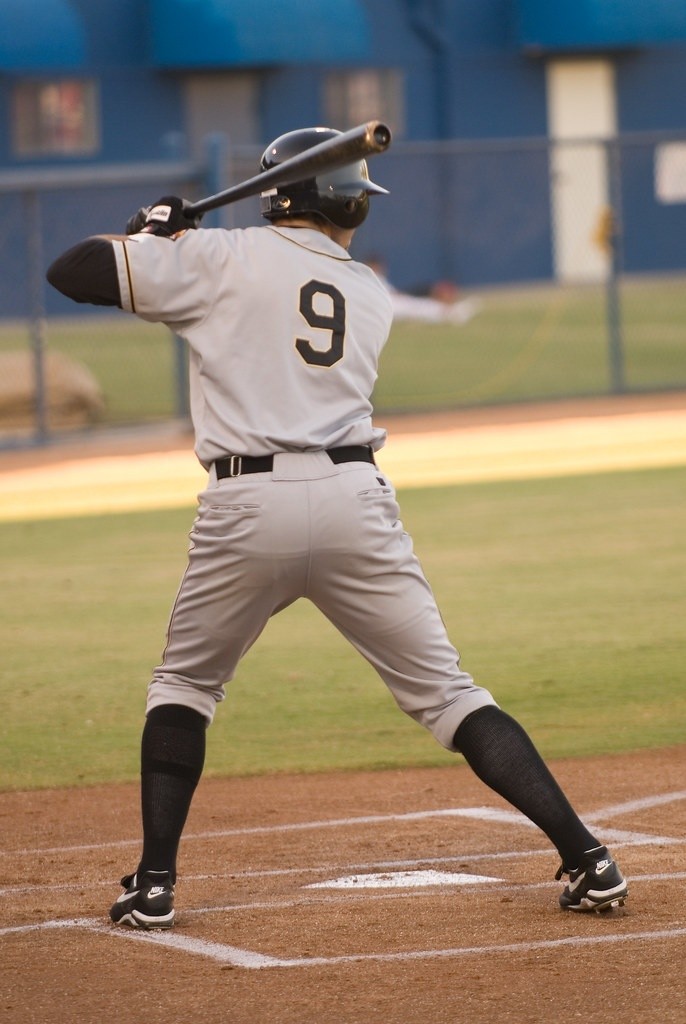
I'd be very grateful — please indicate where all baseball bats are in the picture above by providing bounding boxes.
[186,119,393,214]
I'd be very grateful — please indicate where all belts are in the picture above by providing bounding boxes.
[215,442,376,480]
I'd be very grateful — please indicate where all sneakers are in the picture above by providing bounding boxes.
[108,868,176,929]
[554,844,629,914]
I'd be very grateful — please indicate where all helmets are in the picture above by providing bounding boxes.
[257,126,391,230]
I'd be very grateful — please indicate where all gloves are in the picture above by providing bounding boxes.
[125,195,206,235]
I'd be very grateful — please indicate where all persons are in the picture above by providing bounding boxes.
[44,126,627,926]
